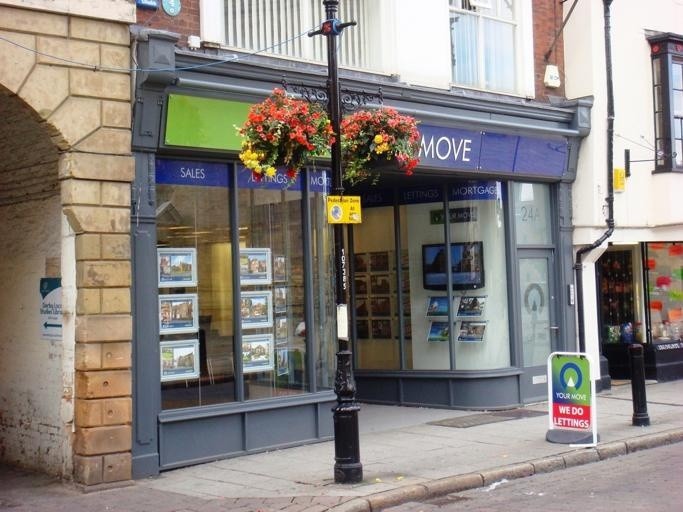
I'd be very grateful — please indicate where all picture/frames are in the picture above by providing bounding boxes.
[273,254,290,377]
[238,249,273,376]
[158,248,200,382]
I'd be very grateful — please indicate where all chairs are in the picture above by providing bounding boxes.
[205,335,235,384]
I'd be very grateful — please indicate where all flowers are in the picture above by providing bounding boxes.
[236,87,418,192]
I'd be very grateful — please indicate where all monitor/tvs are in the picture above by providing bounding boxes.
[422,241,485,291]
